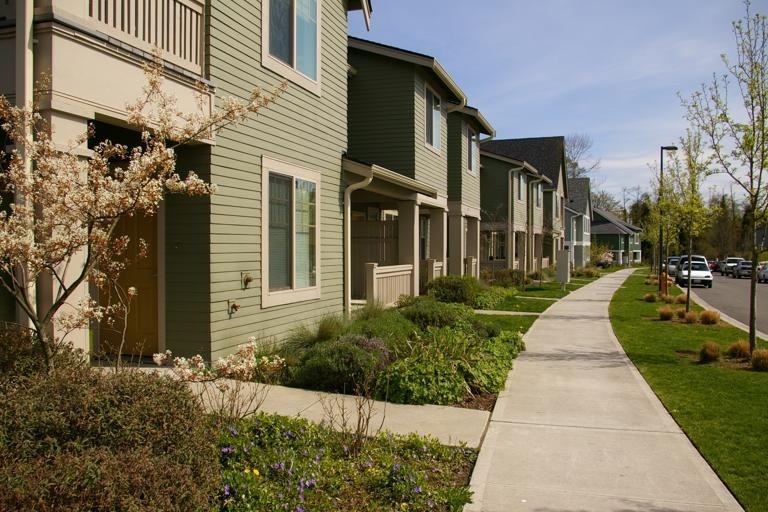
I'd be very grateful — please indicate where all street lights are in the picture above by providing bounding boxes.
[657,144,677,295]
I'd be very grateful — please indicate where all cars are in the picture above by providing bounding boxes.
[660,255,766,287]
